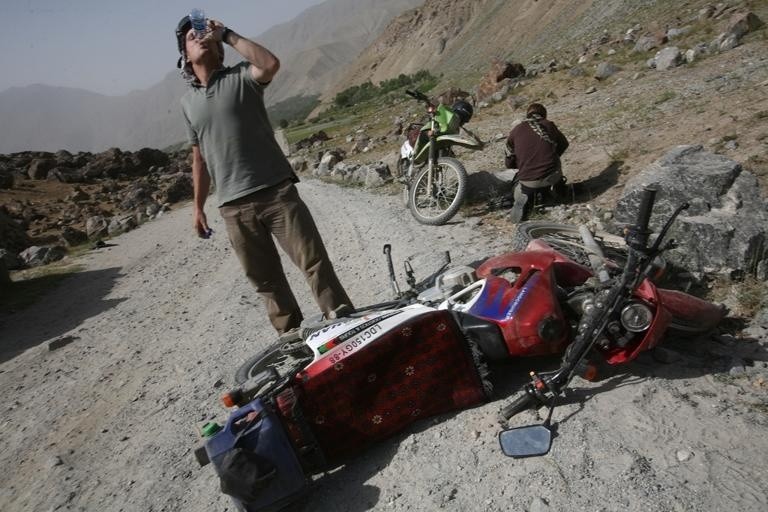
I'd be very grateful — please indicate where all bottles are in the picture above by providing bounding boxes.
[188,7,207,39]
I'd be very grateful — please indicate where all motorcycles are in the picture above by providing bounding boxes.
[192,185,731,512]
[394,89,484,227]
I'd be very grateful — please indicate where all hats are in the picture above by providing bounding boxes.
[451,101,473,122]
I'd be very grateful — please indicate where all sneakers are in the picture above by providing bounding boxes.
[509,193,528,223]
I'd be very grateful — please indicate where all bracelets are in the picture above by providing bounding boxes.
[221,28,234,45]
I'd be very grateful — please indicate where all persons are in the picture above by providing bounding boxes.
[175,7,356,336]
[502,102,571,225]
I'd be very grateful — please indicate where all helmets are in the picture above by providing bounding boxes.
[526,104,547,117]
[177,13,224,66]
